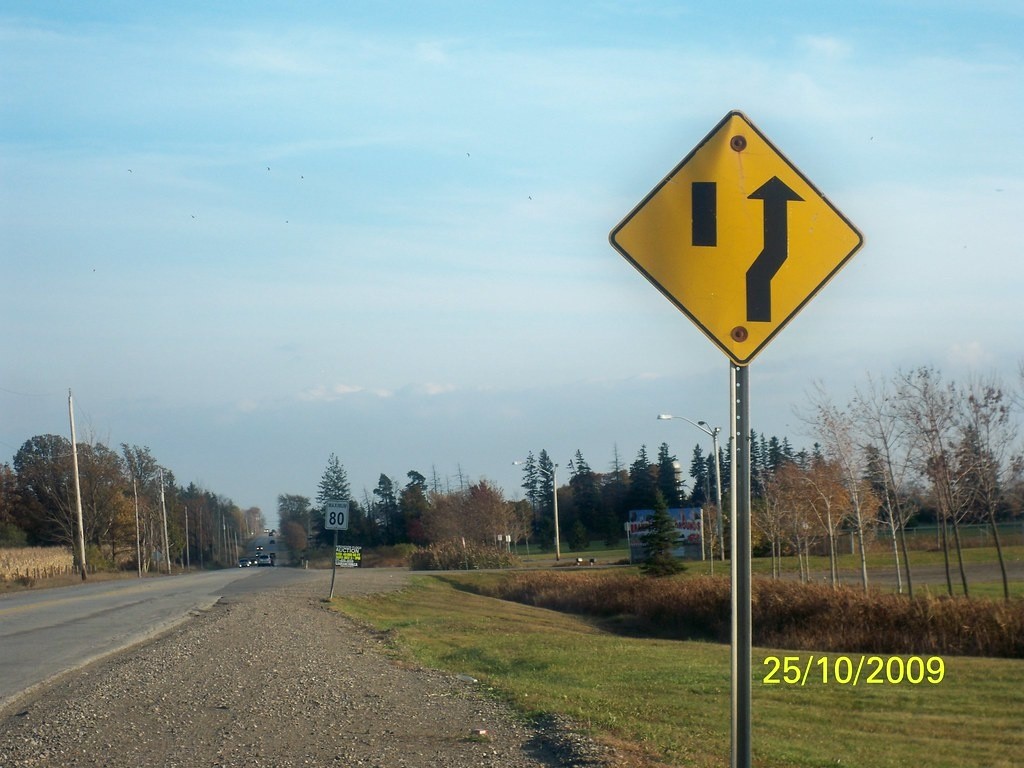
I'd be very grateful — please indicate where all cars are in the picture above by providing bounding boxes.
[239,546,277,568]
[263,529,277,545]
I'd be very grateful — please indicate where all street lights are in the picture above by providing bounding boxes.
[658,412,726,562]
[512,461,561,561]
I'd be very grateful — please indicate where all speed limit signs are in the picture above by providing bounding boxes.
[325,499,349,531]
